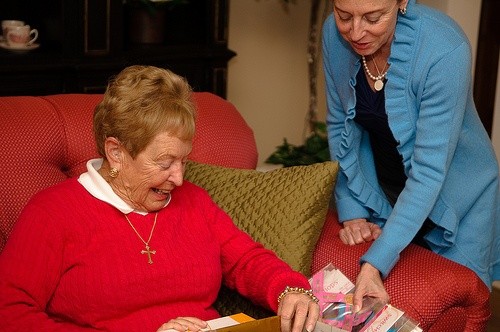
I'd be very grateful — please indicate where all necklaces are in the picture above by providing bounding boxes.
[123,211,158,264]
[362,54,391,92]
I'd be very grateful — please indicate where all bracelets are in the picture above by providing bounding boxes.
[277,285,319,304]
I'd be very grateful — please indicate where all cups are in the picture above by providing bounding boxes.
[6,26,38,46]
[1,19,29,31]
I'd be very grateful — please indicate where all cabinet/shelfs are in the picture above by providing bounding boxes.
[0,0,230,100]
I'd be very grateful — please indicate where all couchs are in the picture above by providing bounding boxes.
[0,92,493,332]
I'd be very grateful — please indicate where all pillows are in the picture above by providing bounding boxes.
[182,160,339,275]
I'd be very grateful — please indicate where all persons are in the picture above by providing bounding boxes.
[0,63,324,332]
[319,0,500,315]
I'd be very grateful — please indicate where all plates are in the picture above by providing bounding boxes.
[0,35,39,52]
